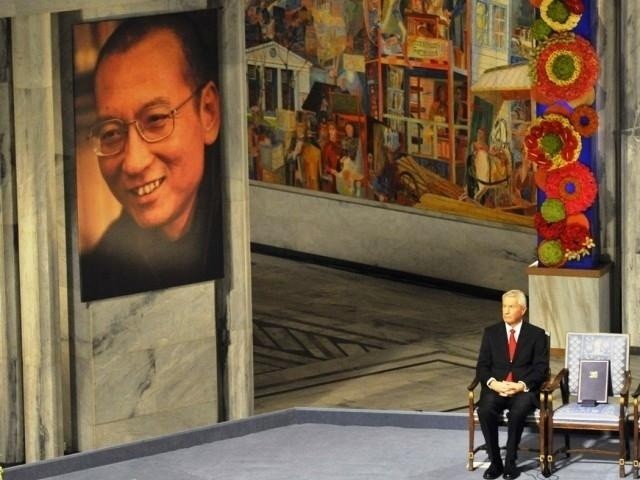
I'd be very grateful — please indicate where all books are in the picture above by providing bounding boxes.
[577,359,610,404]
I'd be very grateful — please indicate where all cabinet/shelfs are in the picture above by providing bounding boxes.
[525,260,614,356]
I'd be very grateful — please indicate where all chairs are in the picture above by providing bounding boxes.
[467,331,551,473]
[544,332,640,479]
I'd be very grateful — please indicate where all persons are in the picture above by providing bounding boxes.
[73,12,222,300]
[341,122,357,158]
[474,289,550,480]
[317,124,328,147]
[284,117,309,187]
[246,121,261,180]
[321,121,341,192]
[318,97,329,123]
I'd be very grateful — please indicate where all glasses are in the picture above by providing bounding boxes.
[86,83,206,157]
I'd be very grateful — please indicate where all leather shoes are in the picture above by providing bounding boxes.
[483,463,520,479]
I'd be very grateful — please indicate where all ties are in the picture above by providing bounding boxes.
[505,329,517,382]
